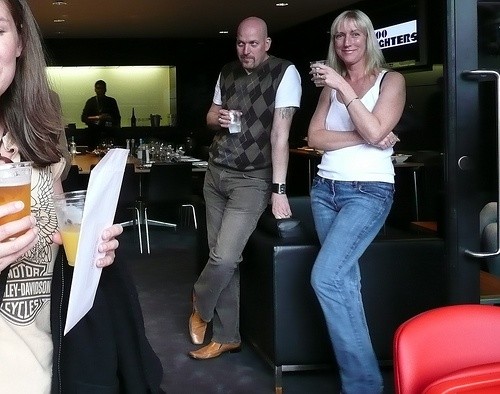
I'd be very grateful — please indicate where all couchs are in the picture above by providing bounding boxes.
[237,193,448,394]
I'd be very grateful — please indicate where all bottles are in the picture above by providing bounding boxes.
[131,107,136,127]
[70,136,76,154]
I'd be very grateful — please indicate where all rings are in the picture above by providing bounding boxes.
[285,214,289,216]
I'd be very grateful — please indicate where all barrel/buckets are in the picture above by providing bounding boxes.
[75,146,88,155]
[150,114,160,127]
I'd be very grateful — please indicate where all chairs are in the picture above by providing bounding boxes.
[392,304,500,394]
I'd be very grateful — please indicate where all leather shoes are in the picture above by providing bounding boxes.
[189,289,207,345]
[189,338,241,360]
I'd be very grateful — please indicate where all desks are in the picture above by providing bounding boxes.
[285,145,425,236]
[67,147,202,233]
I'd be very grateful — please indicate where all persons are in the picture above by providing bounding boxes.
[81,79,121,131]
[0,0,123,394]
[307,10,406,394]
[189,17,302,359]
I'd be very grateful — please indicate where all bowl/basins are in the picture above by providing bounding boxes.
[390,155,411,163]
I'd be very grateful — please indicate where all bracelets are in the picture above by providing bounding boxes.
[271,183,286,194]
[346,98,359,108]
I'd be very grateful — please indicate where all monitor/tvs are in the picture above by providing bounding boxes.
[365,0,428,68]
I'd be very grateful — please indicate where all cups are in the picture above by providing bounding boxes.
[51,190,87,266]
[0,160,34,239]
[226,110,241,134]
[309,60,329,87]
